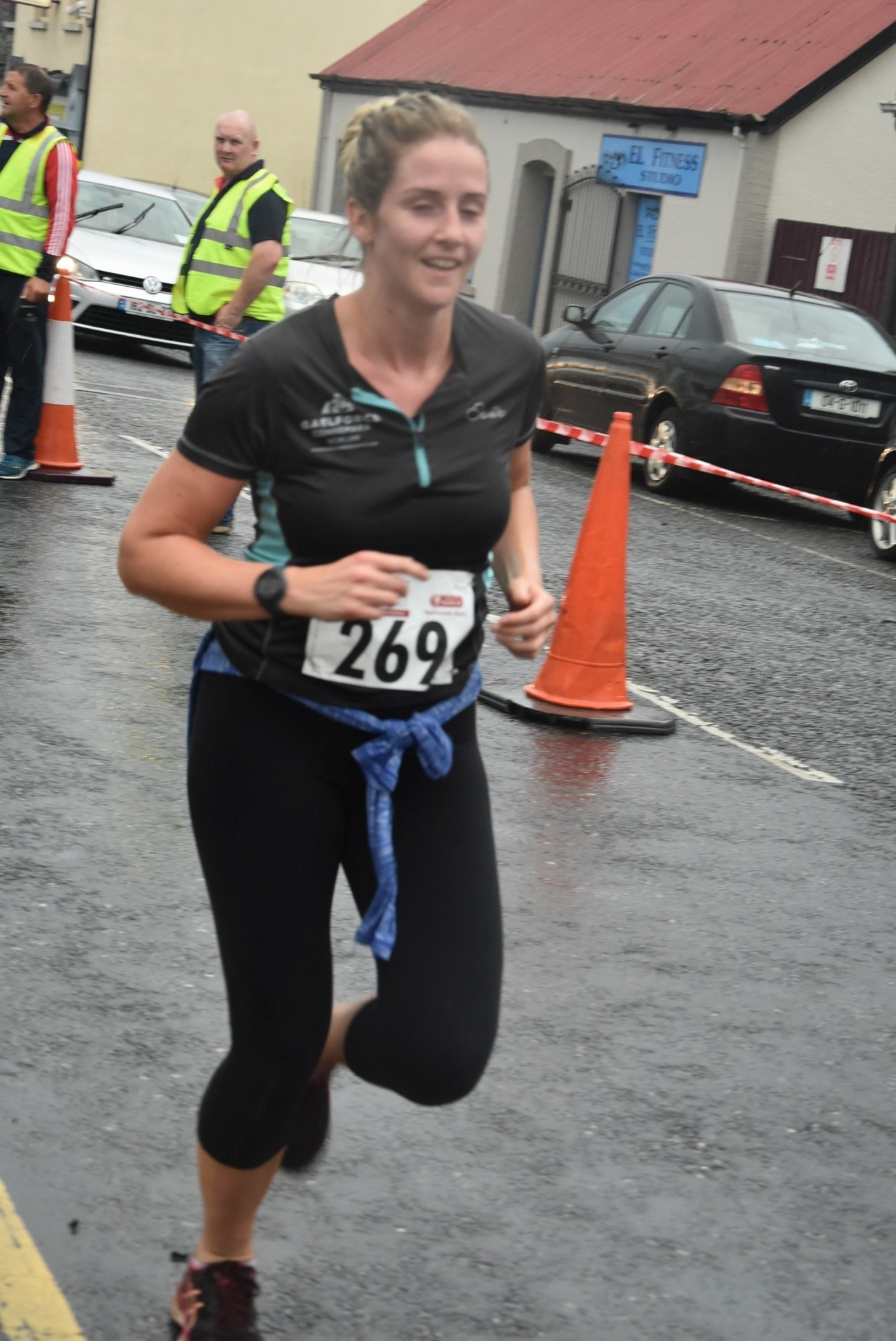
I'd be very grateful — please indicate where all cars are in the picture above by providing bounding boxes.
[536,274,896,561]
[282,208,367,322]
[46,169,212,359]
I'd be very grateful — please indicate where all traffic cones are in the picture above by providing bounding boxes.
[24,277,117,484]
[476,413,676,735]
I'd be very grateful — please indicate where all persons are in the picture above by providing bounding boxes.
[123,88,558,1341]
[171,109,293,536]
[0,63,80,481]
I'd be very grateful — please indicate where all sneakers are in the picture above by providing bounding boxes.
[281,1069,331,1170]
[0,455,40,479]
[169,1258,265,1341]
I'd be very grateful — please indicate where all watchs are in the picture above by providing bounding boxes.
[254,560,296,620]
